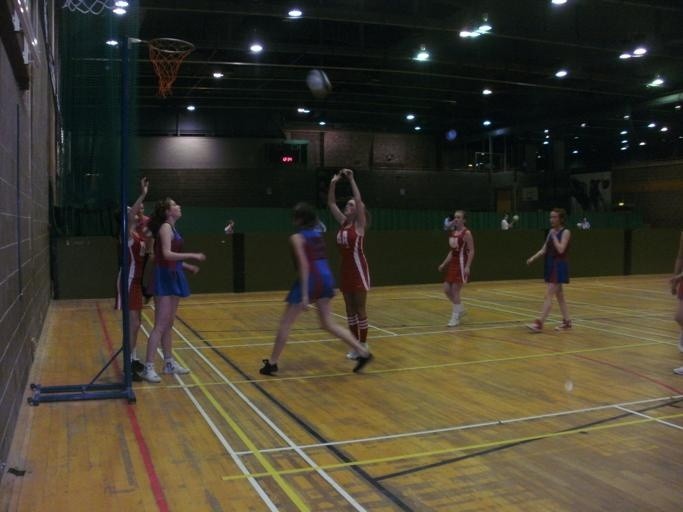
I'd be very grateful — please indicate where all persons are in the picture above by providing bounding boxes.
[500,212,520,232]
[259,200,375,374]
[443,212,457,231]
[112,175,208,383]
[525,207,572,334]
[327,166,373,361]
[668,230,683,375]
[224,220,235,233]
[437,209,475,327]
[579,217,592,231]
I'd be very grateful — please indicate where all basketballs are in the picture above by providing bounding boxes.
[307,67,329,91]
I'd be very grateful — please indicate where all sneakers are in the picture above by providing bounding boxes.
[162,358,189,375]
[526,319,544,332]
[673,366,683,377]
[129,360,160,386]
[448,308,467,327]
[346,340,374,375]
[257,358,278,377]
[555,319,573,332]
